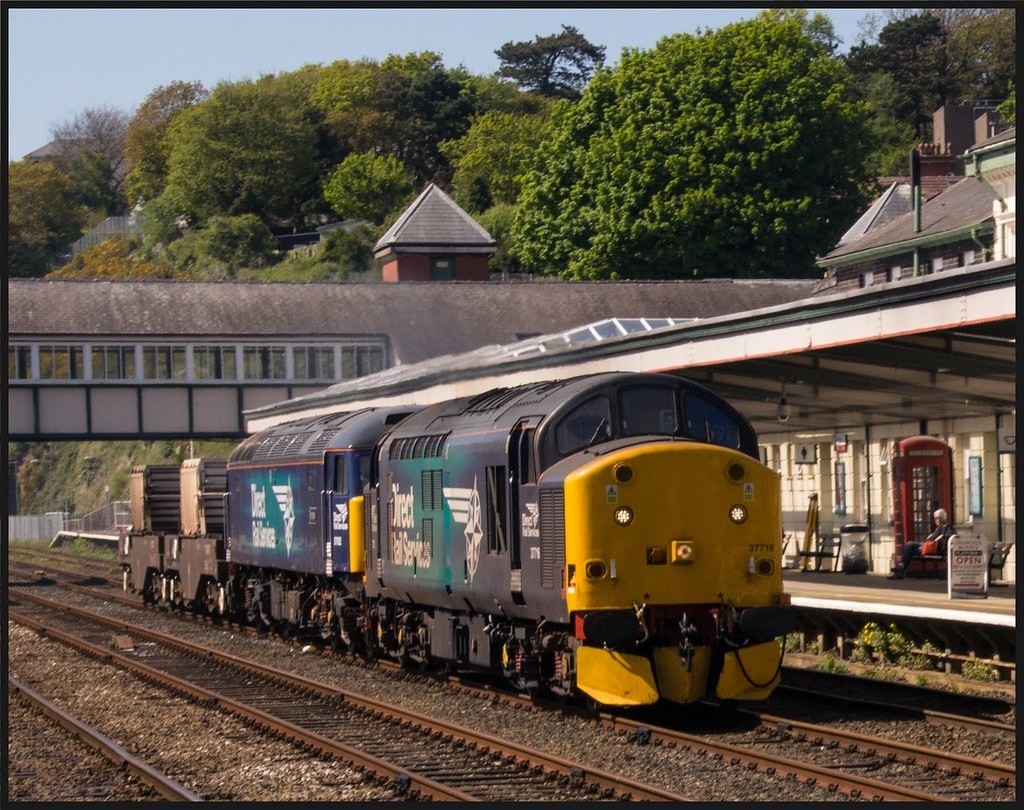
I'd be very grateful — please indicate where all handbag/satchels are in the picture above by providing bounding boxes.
[922,540,938,556]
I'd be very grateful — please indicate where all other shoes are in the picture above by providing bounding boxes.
[890,565,905,573]
[886,573,903,580]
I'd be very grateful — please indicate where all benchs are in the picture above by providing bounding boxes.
[889,542,1014,588]
[799,534,842,573]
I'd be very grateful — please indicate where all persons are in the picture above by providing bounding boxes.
[886,509,956,579]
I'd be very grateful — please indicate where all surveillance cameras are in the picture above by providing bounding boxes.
[778,399,791,422]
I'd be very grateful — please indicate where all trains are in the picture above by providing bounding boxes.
[114,371,792,711]
